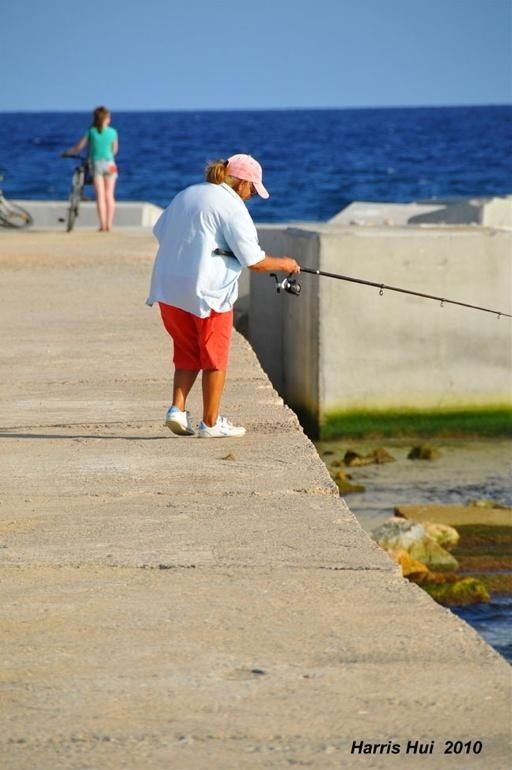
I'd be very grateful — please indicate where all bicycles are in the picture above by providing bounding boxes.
[59,153,92,232]
[0,176,32,229]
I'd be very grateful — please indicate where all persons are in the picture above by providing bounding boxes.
[60,107,119,232]
[144,153,301,439]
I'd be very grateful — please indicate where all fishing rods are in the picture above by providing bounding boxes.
[213,249,512,319]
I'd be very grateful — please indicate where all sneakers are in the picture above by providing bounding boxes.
[199,415,246,436]
[166,405,196,436]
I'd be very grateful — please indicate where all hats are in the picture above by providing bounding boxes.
[224,153,270,200]
